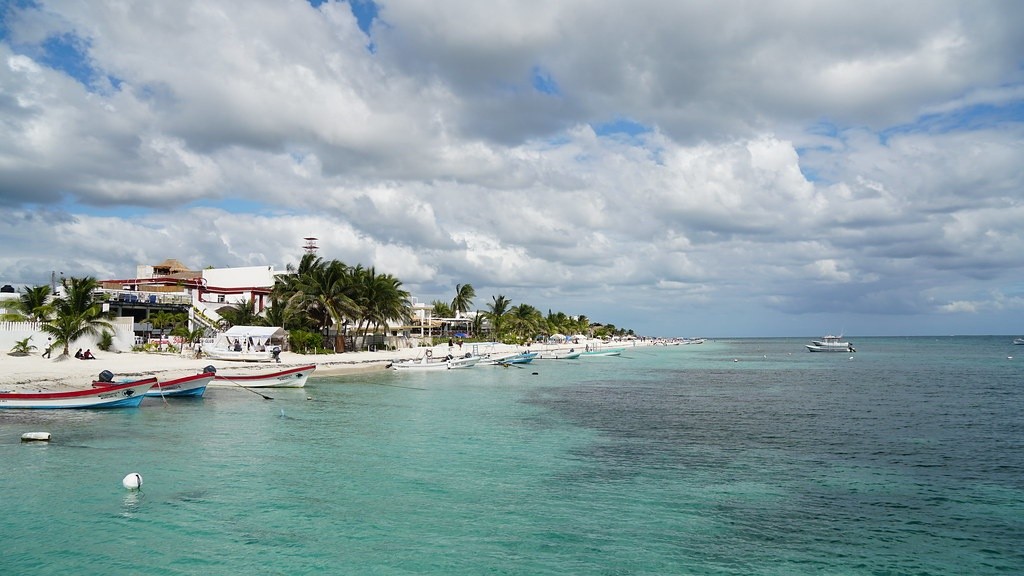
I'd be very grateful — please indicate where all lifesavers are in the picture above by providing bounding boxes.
[425,349,433,358]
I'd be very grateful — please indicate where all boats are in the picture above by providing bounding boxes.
[537,351,580,359]
[1013,338,1024,345]
[0,374,160,409]
[448,355,481,369]
[206,364,317,388]
[91,371,215,399]
[804,335,849,352]
[488,352,538,366]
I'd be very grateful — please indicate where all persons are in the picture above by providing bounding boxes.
[570,348,574,352]
[229,340,250,351]
[524,350,530,358]
[586,344,589,352]
[635,337,700,345]
[42,337,95,360]
[616,339,623,343]
[449,337,462,350]
[192,341,201,359]
[494,337,495,342]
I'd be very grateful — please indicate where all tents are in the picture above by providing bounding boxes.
[225,325,288,346]
[537,333,586,340]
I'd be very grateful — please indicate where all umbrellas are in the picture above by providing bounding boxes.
[454,332,468,339]
[597,335,636,339]
